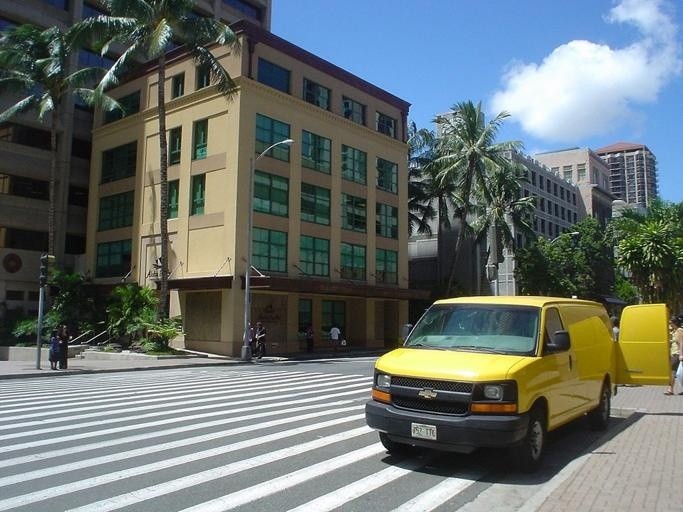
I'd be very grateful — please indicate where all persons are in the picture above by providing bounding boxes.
[241,322,255,357]
[254,321,266,360]
[662,315,682,396]
[328,324,341,342]
[47,328,60,371]
[295,328,304,352]
[305,326,315,354]
[56,324,72,370]
[608,315,620,343]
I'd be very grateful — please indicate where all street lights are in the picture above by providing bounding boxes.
[239,137,296,362]
[551,231,581,243]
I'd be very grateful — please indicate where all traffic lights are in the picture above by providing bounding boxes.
[39,252,56,287]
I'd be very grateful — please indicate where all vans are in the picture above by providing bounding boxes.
[363,293,675,476]
